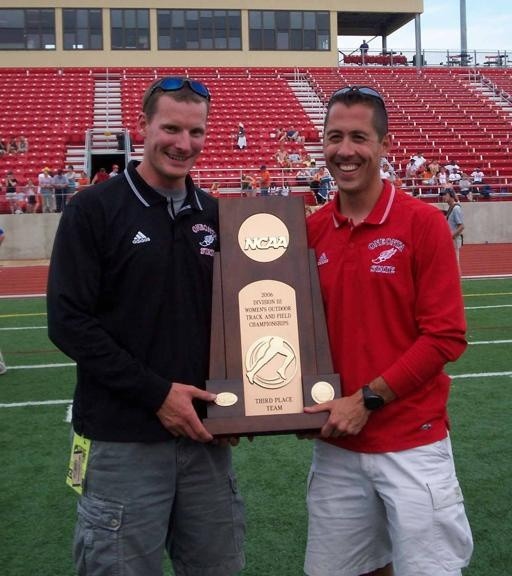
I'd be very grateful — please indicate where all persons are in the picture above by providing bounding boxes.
[437,187,465,265]
[360,40,369,66]
[303,86,474,575]
[46,76,250,576]
[0,227,6,246]
[237,120,485,202]
[0,135,120,214]
[208,180,221,194]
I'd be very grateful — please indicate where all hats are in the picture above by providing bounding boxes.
[439,187,454,197]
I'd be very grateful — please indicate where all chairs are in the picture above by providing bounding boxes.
[0,64,512,214]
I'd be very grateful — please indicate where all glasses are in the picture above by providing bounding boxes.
[331,85,386,110]
[142,76,210,112]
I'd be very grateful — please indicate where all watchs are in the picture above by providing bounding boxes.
[362,383,385,411]
